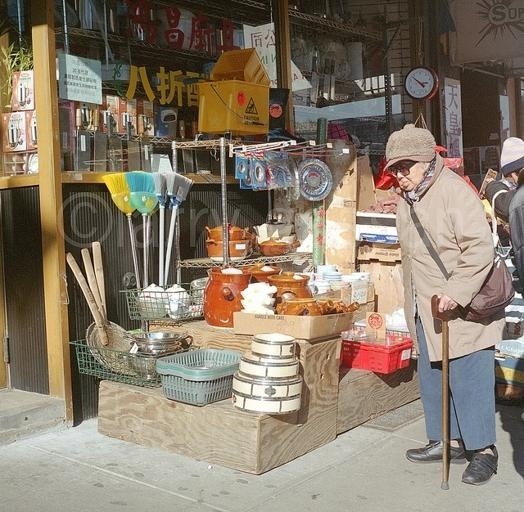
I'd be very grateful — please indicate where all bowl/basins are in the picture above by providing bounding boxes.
[324,273,371,285]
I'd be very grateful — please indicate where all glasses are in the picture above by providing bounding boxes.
[389,162,415,176]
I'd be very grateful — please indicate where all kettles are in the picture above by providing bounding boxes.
[80,104,152,132]
[6,84,38,149]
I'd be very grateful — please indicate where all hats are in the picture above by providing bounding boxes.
[383,123,436,172]
[500,137,524,177]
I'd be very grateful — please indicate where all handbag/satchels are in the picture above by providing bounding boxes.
[460,253,515,321]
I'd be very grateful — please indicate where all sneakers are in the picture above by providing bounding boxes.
[462,444,499,485]
[407,441,466,464]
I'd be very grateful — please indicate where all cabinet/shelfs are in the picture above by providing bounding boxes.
[54,1,394,157]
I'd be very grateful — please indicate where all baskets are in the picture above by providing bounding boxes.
[68,329,241,407]
[118,283,208,322]
[342,336,413,374]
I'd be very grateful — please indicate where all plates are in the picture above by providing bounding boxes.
[210,258,247,262]
[27,153,39,173]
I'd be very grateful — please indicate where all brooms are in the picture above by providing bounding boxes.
[102,168,194,287]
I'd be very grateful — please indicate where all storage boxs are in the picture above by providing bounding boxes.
[495,356,524,387]
[232,310,353,341]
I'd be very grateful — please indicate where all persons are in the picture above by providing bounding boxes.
[501,136,523,299]
[382,123,501,486]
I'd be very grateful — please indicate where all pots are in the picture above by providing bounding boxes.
[116,331,189,381]
[149,7,201,54]
[203,223,290,257]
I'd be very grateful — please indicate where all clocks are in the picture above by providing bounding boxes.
[404,65,440,100]
